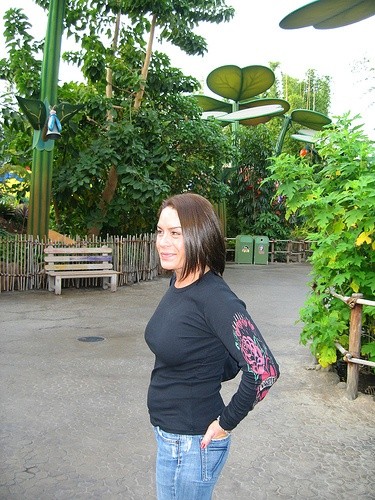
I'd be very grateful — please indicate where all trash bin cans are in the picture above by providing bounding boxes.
[235,235,269,265]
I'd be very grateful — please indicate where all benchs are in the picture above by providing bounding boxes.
[42,245,124,295]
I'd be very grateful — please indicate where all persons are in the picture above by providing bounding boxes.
[143,193,280,500]
